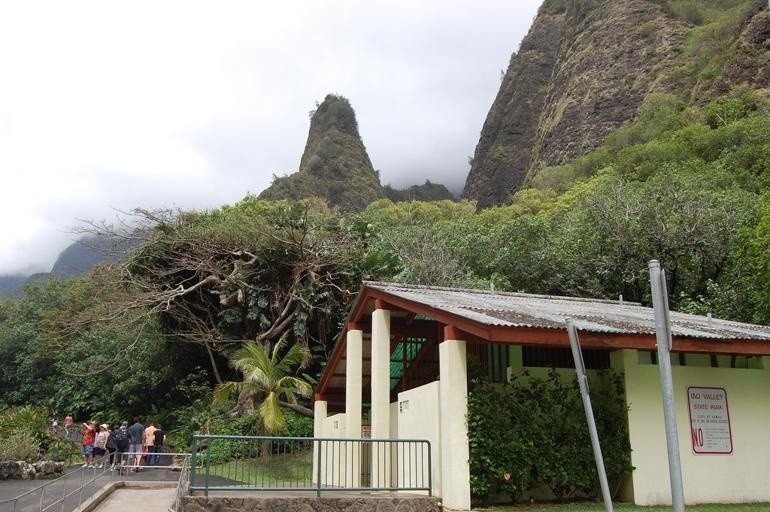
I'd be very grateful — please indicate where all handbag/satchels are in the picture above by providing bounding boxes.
[142,441,149,458]
[128,429,136,443]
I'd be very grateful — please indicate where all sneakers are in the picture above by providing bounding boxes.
[82,462,103,469]
[110,466,143,472]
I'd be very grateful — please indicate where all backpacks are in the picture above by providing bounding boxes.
[112,429,127,441]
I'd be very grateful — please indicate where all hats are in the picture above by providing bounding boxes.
[100,424,108,431]
[112,425,120,429]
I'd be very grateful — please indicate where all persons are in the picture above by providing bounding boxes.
[81,416,166,473]
[63,413,73,436]
[50,413,58,435]
